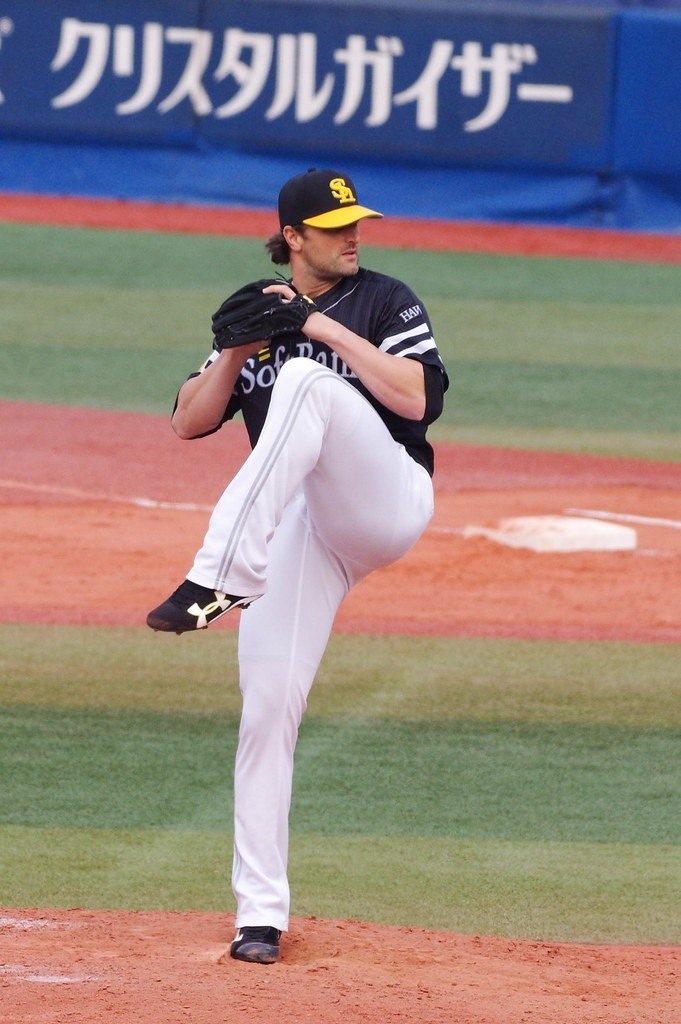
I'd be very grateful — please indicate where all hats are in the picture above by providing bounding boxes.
[279,167,384,229]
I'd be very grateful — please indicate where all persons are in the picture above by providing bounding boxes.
[148,168,450,963]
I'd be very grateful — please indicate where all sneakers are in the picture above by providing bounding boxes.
[146,582,263,635]
[230,926,281,964]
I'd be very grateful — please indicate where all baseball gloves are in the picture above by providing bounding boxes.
[211,271,320,351]
